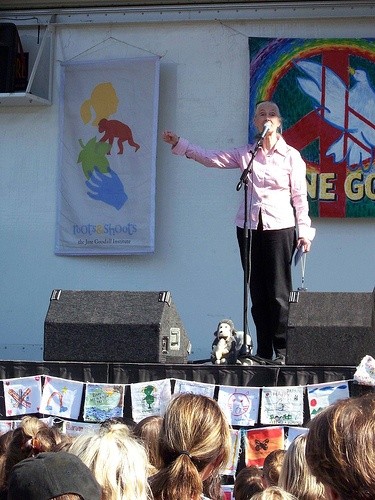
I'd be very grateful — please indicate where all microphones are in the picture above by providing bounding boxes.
[255,122,271,149]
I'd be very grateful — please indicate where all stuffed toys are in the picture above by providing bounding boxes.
[210,319,253,366]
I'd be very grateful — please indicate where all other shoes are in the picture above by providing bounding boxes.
[273,358,285,365]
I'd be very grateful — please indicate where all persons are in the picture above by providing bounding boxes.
[160,101,315,366]
[0,391,374,499]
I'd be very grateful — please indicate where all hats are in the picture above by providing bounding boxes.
[6,451,102,500]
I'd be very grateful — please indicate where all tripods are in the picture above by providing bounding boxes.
[187,143,283,365]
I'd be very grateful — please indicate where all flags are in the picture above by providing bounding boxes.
[54,56,160,256]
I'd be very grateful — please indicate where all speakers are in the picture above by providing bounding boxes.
[0,22,18,93]
[44,289,192,363]
[285,290,375,365]
